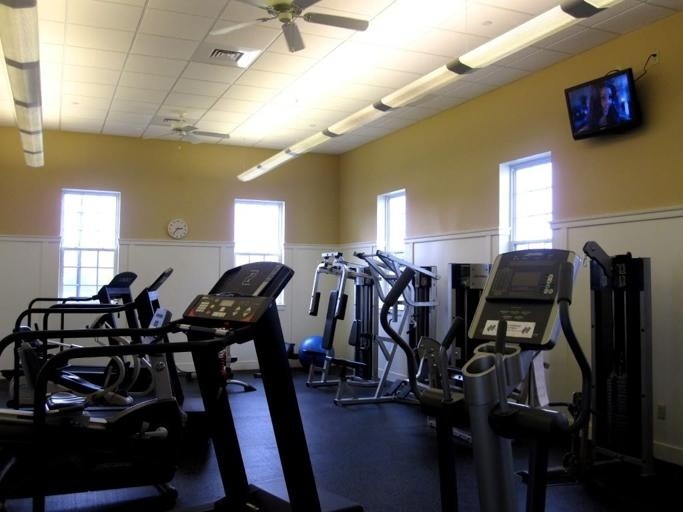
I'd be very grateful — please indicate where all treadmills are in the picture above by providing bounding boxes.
[2,263,365,512]
[1,267,189,473]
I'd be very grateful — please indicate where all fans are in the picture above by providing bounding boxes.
[208,0,369,52]
[142,112,231,143]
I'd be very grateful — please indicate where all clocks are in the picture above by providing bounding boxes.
[167,218,190,239]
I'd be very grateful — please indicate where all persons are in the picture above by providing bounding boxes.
[577,93,593,127]
[573,80,631,137]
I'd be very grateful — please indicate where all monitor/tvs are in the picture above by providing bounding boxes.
[563,68,642,141]
